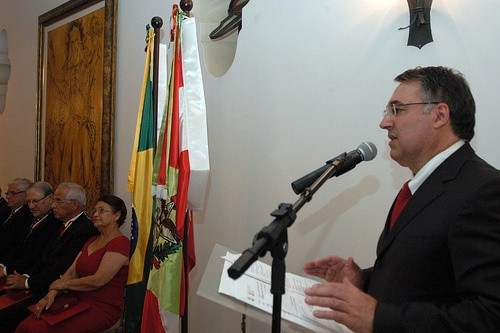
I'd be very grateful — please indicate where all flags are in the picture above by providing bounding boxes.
[122,3,211,333]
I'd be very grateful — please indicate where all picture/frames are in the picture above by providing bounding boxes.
[33,0,119,221]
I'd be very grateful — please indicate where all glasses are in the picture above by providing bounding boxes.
[25,195,47,205]
[384,102,440,117]
[5,191,24,197]
[93,208,112,214]
[53,198,73,205]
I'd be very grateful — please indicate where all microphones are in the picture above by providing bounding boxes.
[291,140,377,195]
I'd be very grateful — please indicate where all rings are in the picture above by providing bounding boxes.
[36,306,39,310]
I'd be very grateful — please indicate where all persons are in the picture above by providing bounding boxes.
[304,65,500,333]
[0,177,131,333]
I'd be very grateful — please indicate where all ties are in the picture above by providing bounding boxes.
[60,221,71,235]
[389,179,412,231]
